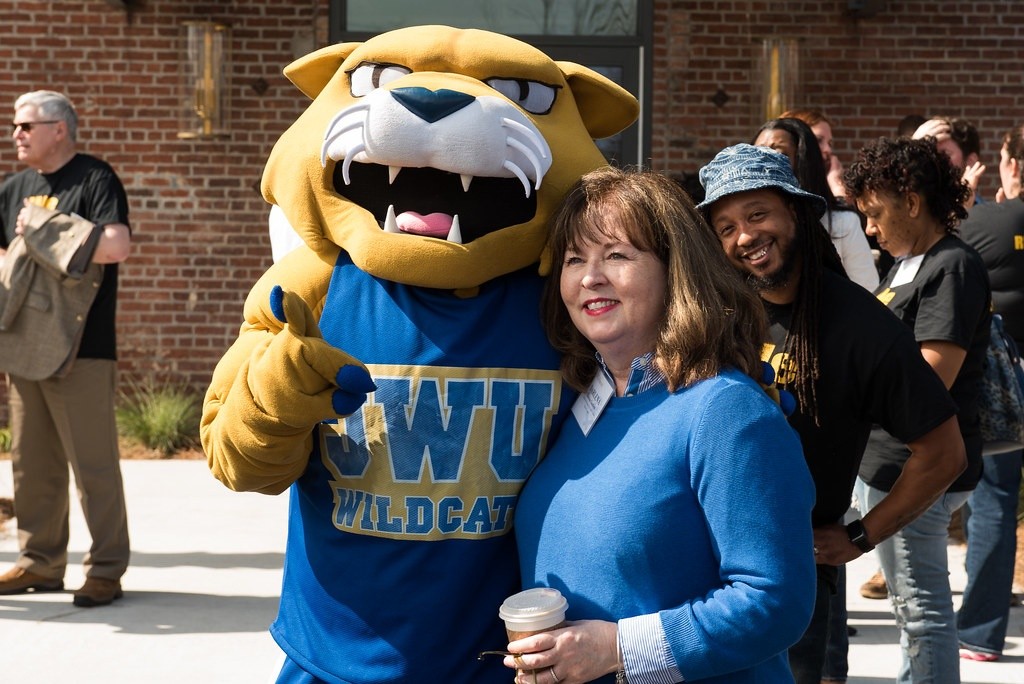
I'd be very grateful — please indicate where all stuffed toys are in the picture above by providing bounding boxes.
[198,23,642,684]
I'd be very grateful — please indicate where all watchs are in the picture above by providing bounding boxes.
[848,520,875,554]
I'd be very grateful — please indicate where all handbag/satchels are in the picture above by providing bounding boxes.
[976,314,1024,457]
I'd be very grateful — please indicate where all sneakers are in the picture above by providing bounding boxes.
[0,565,64,595]
[73,575,124,607]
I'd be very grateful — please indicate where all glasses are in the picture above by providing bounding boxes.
[12,119,57,132]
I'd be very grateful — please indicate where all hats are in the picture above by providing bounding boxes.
[693,143,827,221]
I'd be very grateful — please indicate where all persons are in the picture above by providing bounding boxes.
[751,118,879,293]
[897,115,927,138]
[694,136,967,684]
[0,90,132,607]
[502,168,816,684]
[952,123,1024,660]
[861,116,987,599]
[780,108,857,212]
[840,135,991,684]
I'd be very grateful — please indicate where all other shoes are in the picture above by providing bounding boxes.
[957,642,1000,662]
[860,573,888,599]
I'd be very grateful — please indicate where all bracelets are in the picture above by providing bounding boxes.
[615,628,624,683]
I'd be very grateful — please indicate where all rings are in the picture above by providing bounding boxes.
[550,668,559,683]
[814,547,819,554]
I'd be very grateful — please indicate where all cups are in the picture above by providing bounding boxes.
[499,587,568,675]
